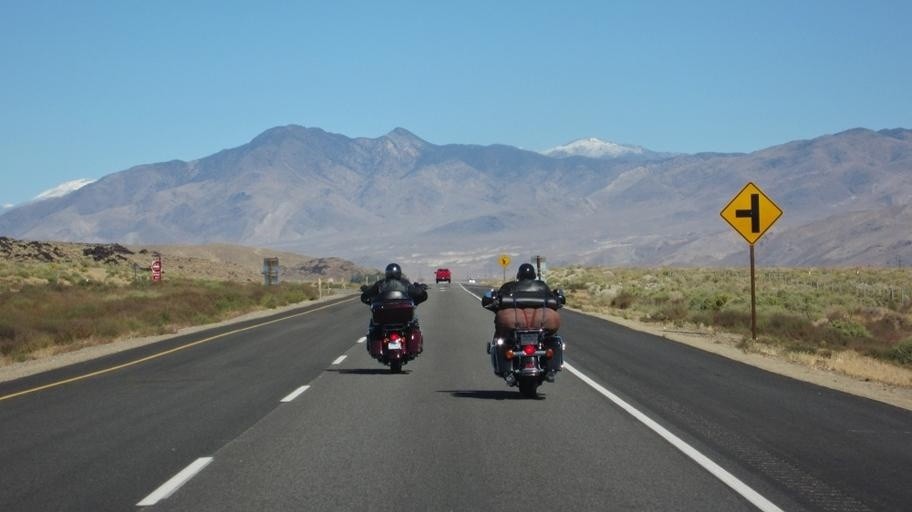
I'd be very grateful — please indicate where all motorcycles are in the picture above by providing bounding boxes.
[360,283,426,372]
[482,289,566,398]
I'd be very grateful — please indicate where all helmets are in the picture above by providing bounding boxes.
[385,263,402,277]
[517,263,537,279]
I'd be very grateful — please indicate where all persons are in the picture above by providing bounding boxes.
[361,262,429,353]
[480,262,565,382]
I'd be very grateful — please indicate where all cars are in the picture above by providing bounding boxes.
[434,269,453,283]
